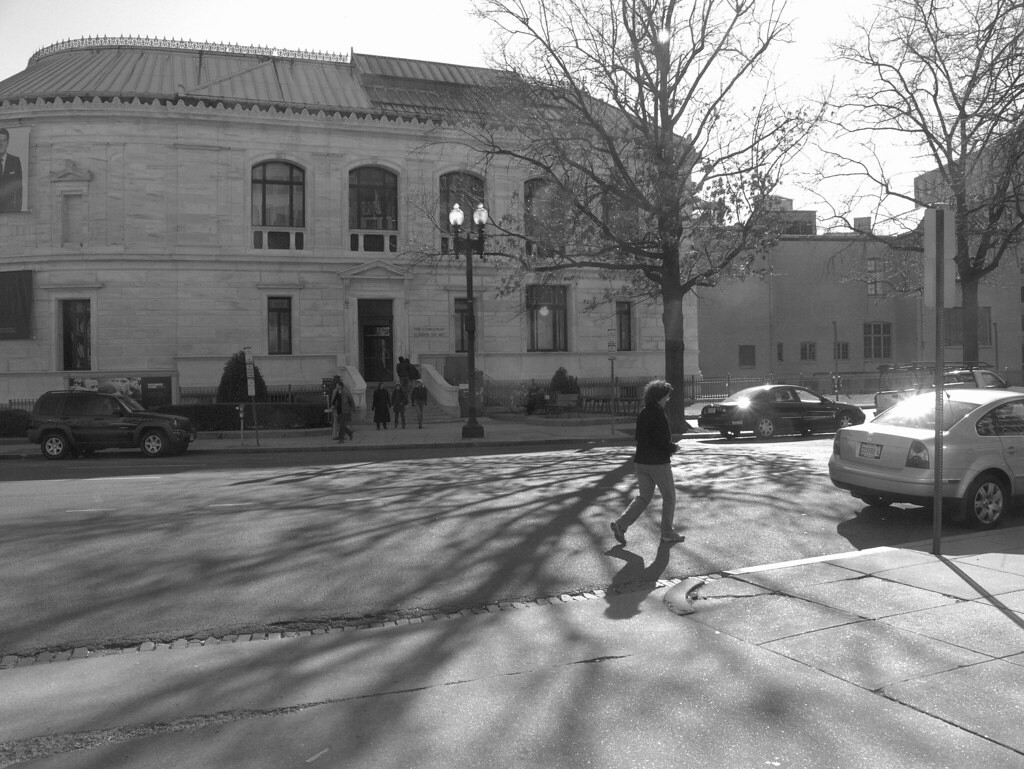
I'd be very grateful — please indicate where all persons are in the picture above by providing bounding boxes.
[610,379,685,545]
[411,379,428,428]
[391,384,409,429]
[331,381,356,444]
[372,383,391,430]
[105,381,121,410]
[396,356,421,404]
[525,378,540,415]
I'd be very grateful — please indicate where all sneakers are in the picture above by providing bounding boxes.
[660,534,686,542]
[610,522,626,544]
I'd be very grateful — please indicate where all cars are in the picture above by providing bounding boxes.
[698,385,867,441]
[827,388,1024,532]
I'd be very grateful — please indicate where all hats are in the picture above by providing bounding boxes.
[417,379,423,385]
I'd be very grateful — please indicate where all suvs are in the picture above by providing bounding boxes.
[27,389,197,460]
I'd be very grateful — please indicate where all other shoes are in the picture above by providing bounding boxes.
[338,442,344,444]
[349,432,353,440]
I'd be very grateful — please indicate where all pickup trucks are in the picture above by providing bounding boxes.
[873,360,1008,417]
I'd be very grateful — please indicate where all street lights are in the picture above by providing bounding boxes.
[448,202,488,440]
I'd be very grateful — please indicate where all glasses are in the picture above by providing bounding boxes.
[665,396,670,402]
